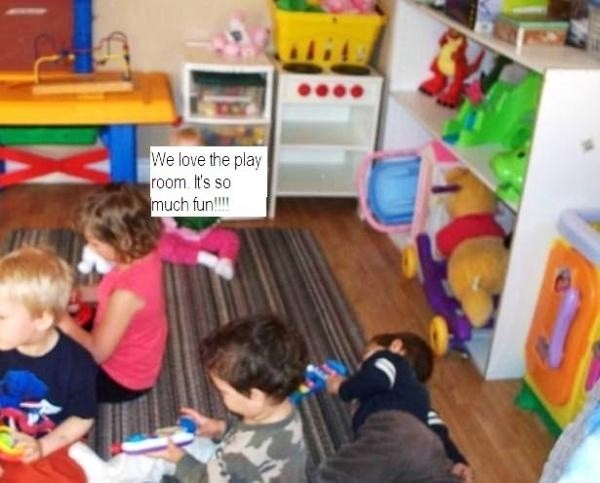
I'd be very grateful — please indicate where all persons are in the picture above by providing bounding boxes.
[55,181,171,405]
[67,308,310,482]
[153,126,244,283]
[76,243,110,277]
[312,326,475,482]
[0,238,103,482]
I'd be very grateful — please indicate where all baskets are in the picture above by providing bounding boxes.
[266,0,387,68]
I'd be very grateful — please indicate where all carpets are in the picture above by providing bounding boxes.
[1,228,373,483]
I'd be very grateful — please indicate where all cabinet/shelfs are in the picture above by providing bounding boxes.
[167,44,274,146]
[372,1,600,380]
[268,52,385,227]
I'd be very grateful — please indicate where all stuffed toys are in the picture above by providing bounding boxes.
[360,0,600,354]
